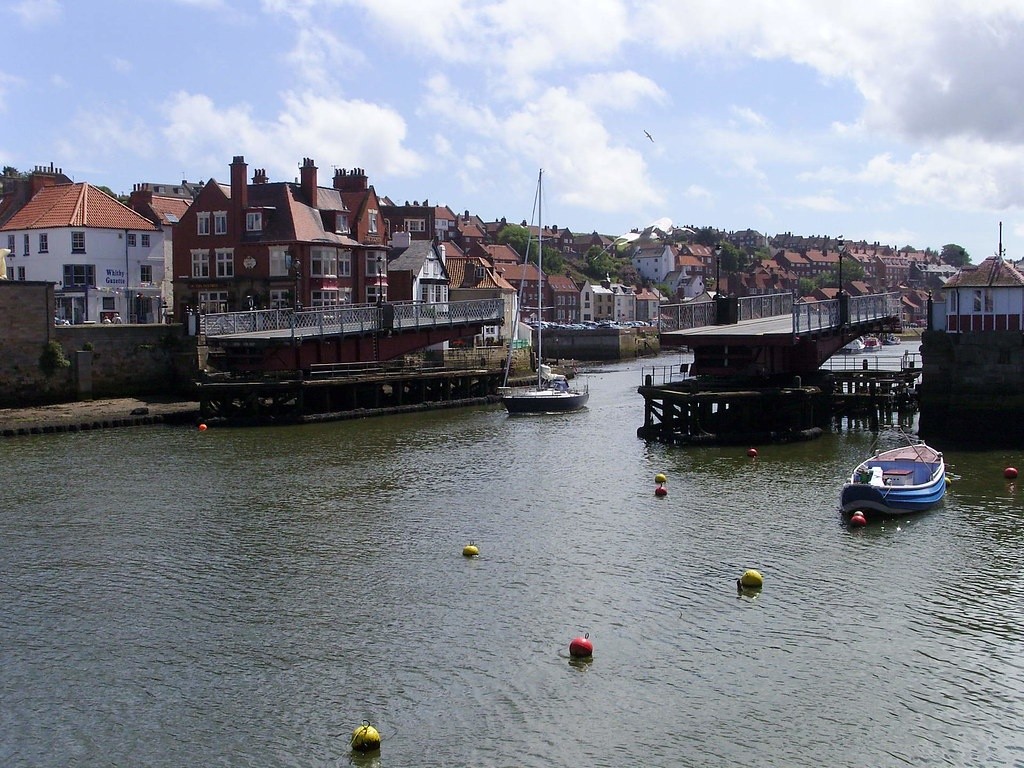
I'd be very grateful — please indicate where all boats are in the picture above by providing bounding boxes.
[840,424,946,525]
[843,340,865,354]
[864,337,883,351]
[770,426,822,442]
[883,335,902,345]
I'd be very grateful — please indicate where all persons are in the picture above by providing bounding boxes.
[102,316,112,324]
[112,313,122,324]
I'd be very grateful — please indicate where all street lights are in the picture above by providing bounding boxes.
[836,236,844,295]
[377,256,384,328]
[312,238,338,308]
[293,258,302,312]
[714,242,723,301]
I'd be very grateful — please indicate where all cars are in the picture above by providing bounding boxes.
[909,322,918,328]
[528,320,649,328]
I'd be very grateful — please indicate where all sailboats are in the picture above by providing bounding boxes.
[499,164,590,411]
